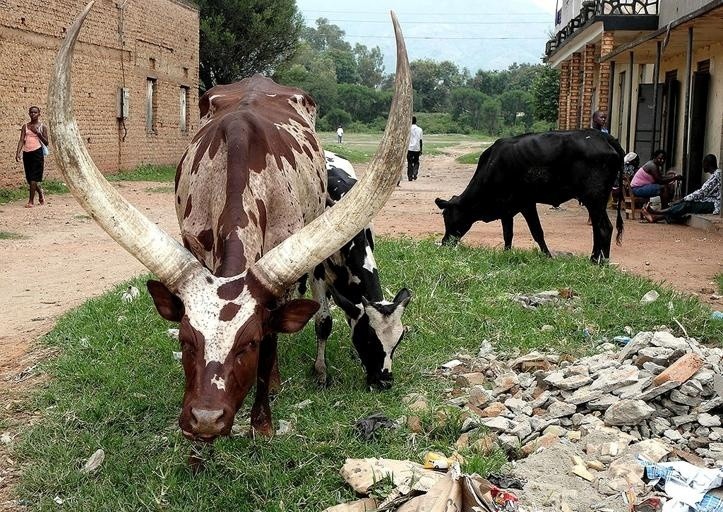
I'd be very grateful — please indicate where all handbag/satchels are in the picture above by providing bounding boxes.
[42,142,49,156]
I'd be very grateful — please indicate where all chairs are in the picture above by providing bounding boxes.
[623,174,650,219]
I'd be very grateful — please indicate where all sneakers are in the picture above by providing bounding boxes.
[25,194,45,209]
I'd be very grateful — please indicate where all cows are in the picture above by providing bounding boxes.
[44,1,416,471]
[432,129,625,265]
[291,144,412,393]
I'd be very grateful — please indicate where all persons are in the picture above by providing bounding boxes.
[589,110,609,133]
[406,116,422,181]
[629,149,684,207]
[14,106,48,207]
[612,152,640,209]
[336,124,343,144]
[642,154,722,224]
